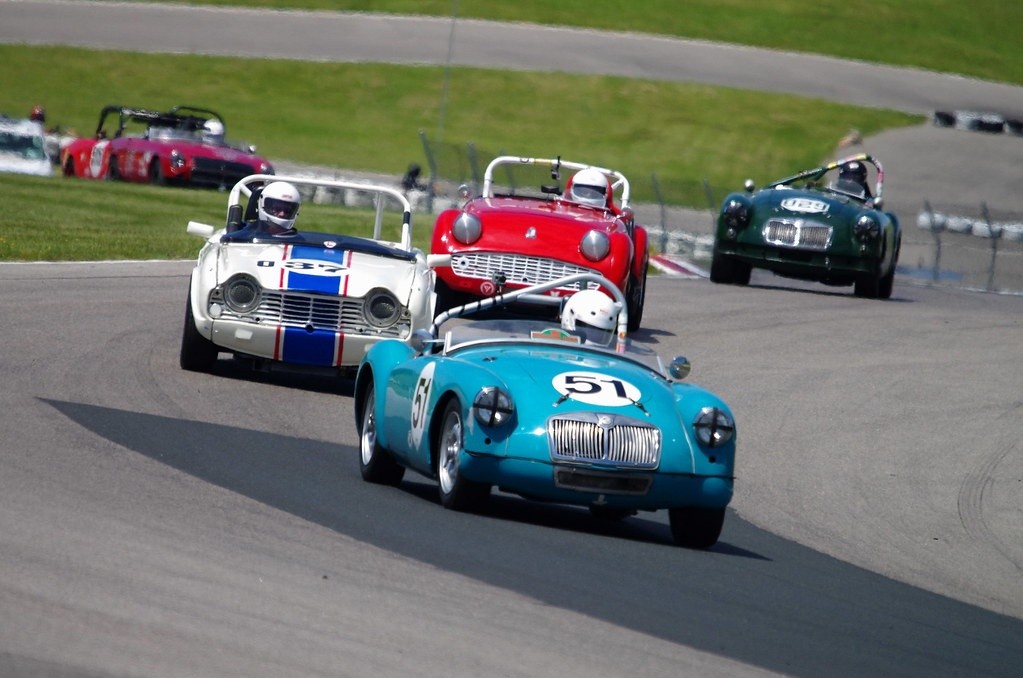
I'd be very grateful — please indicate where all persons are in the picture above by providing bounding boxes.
[837,159,869,194]
[29,105,46,123]
[571,168,610,209]
[255,181,303,230]
[201,119,227,145]
[561,289,617,349]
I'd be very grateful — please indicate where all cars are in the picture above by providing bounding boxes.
[60,104,276,192]
[0,118,51,178]
[709,153,903,298]
[44,127,78,166]
[349,271,737,547]
[428,155,650,335]
[178,174,437,386]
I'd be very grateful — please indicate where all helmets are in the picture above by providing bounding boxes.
[202,119,224,136]
[570,169,608,208]
[560,289,617,348]
[840,161,867,183]
[258,181,301,230]
